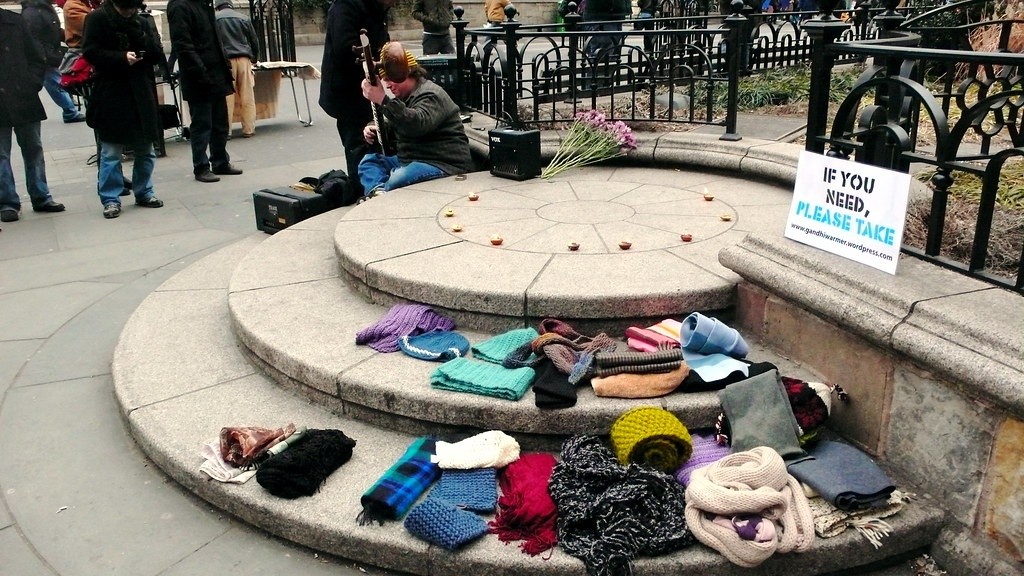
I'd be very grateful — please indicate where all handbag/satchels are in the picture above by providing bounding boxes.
[58,48,95,96]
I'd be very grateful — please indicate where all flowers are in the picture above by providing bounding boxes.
[540,111,636,181]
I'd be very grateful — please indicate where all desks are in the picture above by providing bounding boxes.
[78,61,323,161]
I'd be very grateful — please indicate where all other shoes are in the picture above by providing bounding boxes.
[1,210,18,221]
[244,134,252,137]
[119,188,129,195]
[227,136,231,140]
[64,113,85,123]
[195,171,220,182]
[123,177,133,189]
[212,165,242,174]
[34,201,65,212]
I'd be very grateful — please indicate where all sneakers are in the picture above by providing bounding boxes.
[104,204,120,218]
[136,196,163,207]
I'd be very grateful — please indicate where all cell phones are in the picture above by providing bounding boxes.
[133,50,147,60]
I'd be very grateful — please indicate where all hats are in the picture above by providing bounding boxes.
[378,43,417,77]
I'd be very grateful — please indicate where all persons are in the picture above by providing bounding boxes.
[214,0,261,140]
[633,0,956,30]
[557,0,633,92]
[356,40,473,205]
[166,0,243,182]
[482,0,521,56]
[0,0,172,223]
[411,0,457,56]
[318,0,393,204]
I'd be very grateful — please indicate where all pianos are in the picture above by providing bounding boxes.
[350,26,392,157]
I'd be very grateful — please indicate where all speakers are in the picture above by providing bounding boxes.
[487,127,541,182]
[252,188,324,234]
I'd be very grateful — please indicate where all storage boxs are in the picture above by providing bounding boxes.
[252,185,322,234]
